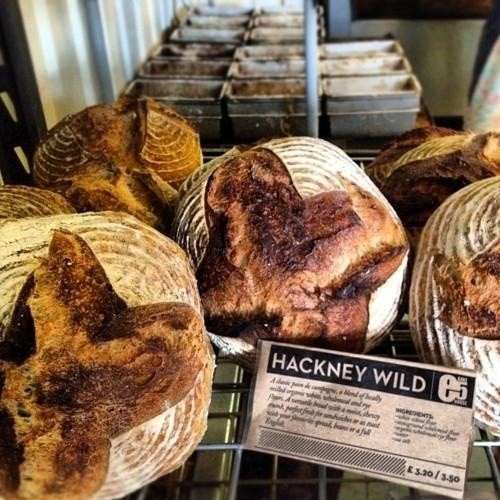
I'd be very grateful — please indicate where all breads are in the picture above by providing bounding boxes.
[0,93,500,500]
